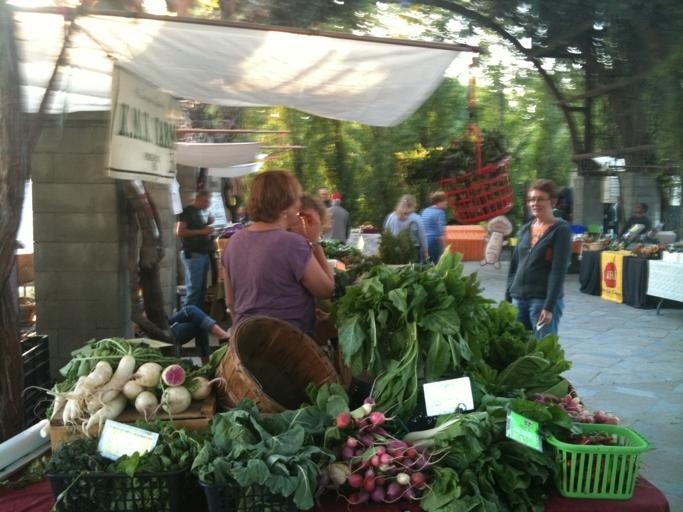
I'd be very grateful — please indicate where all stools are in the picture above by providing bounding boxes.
[159,342,184,358]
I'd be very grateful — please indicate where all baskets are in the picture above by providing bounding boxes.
[539,418,651,501]
[435,124,518,227]
[42,444,198,512]
[198,439,327,512]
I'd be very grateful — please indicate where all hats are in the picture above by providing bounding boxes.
[329,192,343,200]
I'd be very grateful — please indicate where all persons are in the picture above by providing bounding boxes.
[615,203,651,246]
[223,170,338,349]
[383,193,429,264]
[134,185,352,359]
[505,180,572,345]
[420,190,449,265]
[551,196,572,221]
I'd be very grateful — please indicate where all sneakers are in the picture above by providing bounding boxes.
[218,335,231,345]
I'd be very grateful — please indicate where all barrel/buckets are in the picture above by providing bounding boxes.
[211,314,341,421]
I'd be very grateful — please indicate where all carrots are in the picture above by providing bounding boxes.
[527,393,620,425]
[336,398,431,505]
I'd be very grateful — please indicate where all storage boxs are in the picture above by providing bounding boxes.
[47,391,218,453]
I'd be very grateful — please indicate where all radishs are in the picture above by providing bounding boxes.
[50,354,210,431]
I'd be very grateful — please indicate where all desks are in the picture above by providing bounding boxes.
[645,250,683,316]
[1,457,670,511]
[578,249,647,308]
[346,232,382,257]
